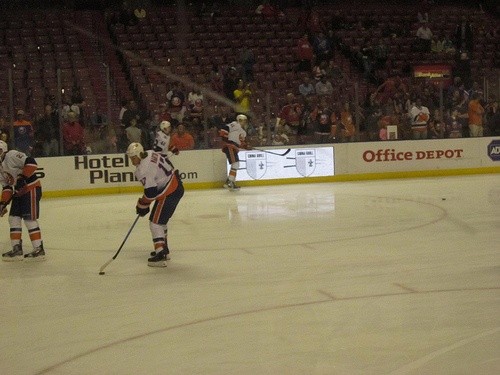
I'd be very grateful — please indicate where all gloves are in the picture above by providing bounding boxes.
[222,140,230,149]
[246,143,251,150]
[170,145,179,156]
[0,205,7,217]
[14,179,25,190]
[136,198,150,217]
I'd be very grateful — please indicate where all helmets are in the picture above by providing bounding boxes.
[160,121,170,130]
[0,140,7,152]
[127,143,145,159]
[237,114,247,121]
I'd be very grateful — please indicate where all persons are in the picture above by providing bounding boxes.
[218,114,251,190]
[0,139,47,262]
[127,142,185,267]
[410,98,430,139]
[468,93,485,137]
[0,0,500,159]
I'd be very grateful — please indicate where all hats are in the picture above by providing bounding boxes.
[17,110,24,115]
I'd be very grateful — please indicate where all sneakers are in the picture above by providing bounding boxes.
[224,179,235,192]
[2,239,24,261]
[24,240,46,263]
[148,249,167,267]
[151,246,170,260]
[232,185,241,191]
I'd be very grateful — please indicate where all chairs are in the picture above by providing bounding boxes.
[0,0,500,118]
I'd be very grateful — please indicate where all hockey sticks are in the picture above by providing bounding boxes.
[1,194,15,215]
[100,214,139,272]
[250,146,291,156]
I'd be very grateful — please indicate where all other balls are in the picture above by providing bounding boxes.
[99,272,105,275]
